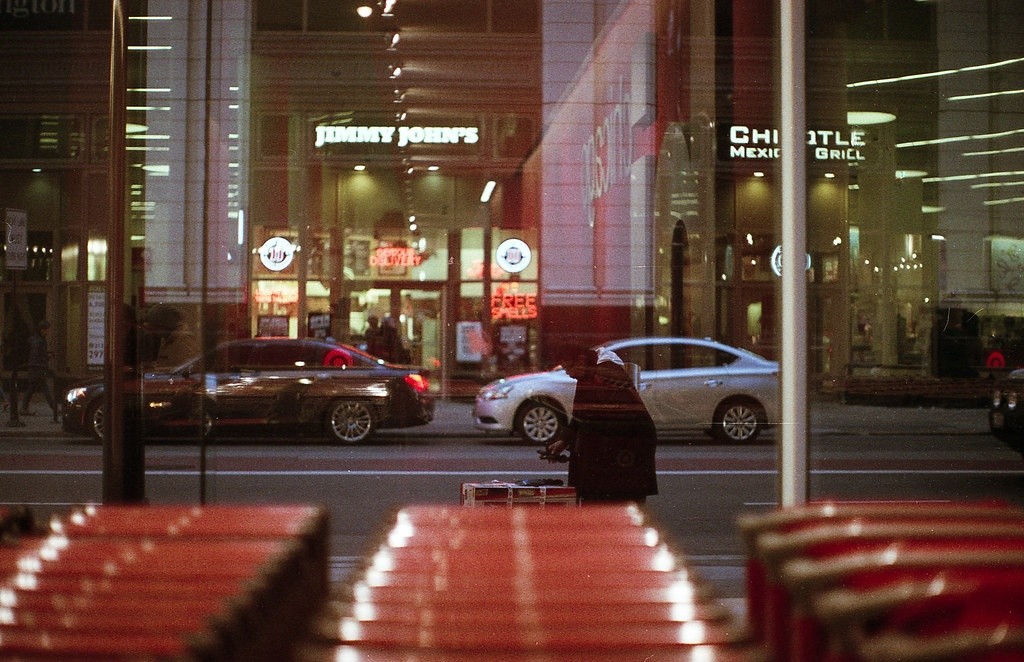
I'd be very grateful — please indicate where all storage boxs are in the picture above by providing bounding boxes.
[459,482,578,509]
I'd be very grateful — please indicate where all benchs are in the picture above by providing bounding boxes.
[52,375,104,425]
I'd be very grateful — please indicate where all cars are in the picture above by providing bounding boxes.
[60,336,435,446]
[470,336,780,446]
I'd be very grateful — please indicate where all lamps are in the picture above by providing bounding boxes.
[392,89,406,104]
[377,0,400,17]
[395,111,407,122]
[383,32,400,51]
[387,64,402,79]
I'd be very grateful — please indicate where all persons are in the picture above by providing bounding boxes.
[19,320,62,416]
[547,344,657,504]
[364,314,400,364]
